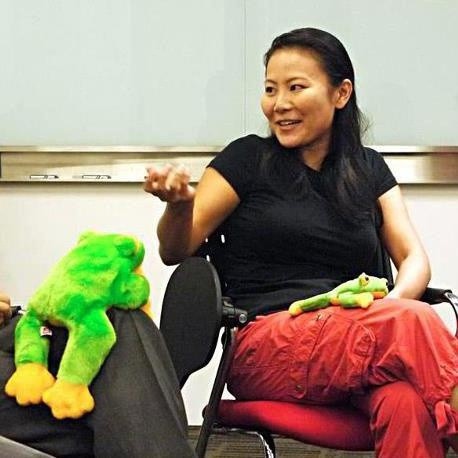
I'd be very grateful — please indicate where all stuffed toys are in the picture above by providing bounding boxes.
[5,231,150,420]
[289,272,389,315]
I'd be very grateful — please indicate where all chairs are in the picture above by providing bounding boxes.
[193,240,458,458]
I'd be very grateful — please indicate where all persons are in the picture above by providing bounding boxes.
[144,28,458,458]
[0,290,198,458]
